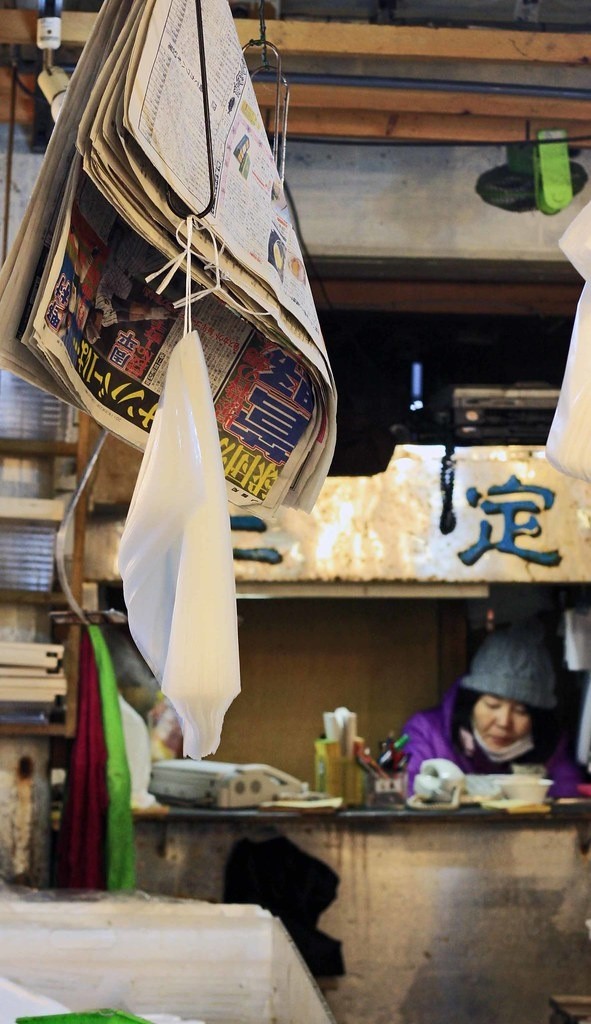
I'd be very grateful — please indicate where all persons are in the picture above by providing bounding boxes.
[85,263,178,346]
[398,627,586,796]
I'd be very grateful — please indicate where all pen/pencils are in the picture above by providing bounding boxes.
[348,731,417,809]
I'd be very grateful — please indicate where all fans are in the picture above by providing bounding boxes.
[476,128,587,213]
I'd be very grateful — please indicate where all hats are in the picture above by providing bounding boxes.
[461,628,557,709]
